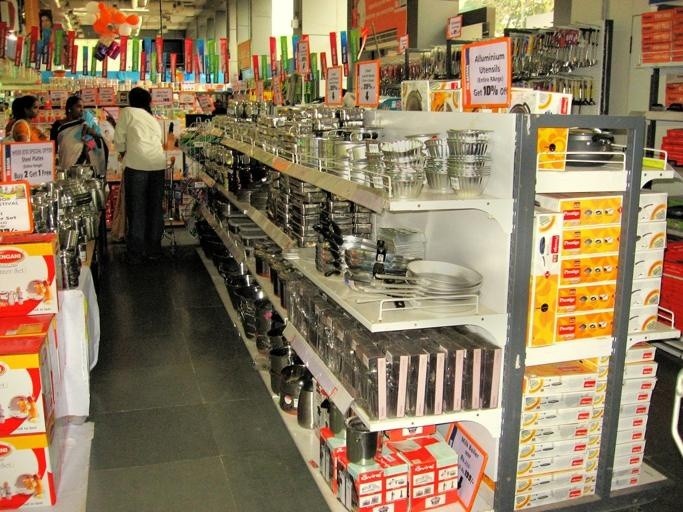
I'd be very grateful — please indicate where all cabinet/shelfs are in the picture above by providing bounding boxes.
[190,0,682,512]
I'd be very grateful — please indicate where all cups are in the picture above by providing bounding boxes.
[330,400,349,434]
[344,414,378,466]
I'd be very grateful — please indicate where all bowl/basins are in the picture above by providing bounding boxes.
[365,129,493,199]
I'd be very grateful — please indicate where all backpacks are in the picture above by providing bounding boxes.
[3,131,15,141]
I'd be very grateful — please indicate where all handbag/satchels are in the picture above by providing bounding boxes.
[111,168,128,242]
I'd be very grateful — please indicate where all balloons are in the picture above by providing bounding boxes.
[80,1,138,39]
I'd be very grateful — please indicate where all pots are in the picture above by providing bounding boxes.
[210,235,296,418]
[566,127,616,167]
[312,211,483,315]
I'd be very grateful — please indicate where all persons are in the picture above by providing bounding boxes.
[49,97,109,265]
[3,94,39,142]
[111,87,168,261]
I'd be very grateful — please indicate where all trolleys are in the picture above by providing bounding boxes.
[111,157,181,257]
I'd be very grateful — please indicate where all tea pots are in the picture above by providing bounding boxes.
[297,364,316,430]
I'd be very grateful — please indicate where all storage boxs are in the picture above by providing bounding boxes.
[1,182,61,512]
